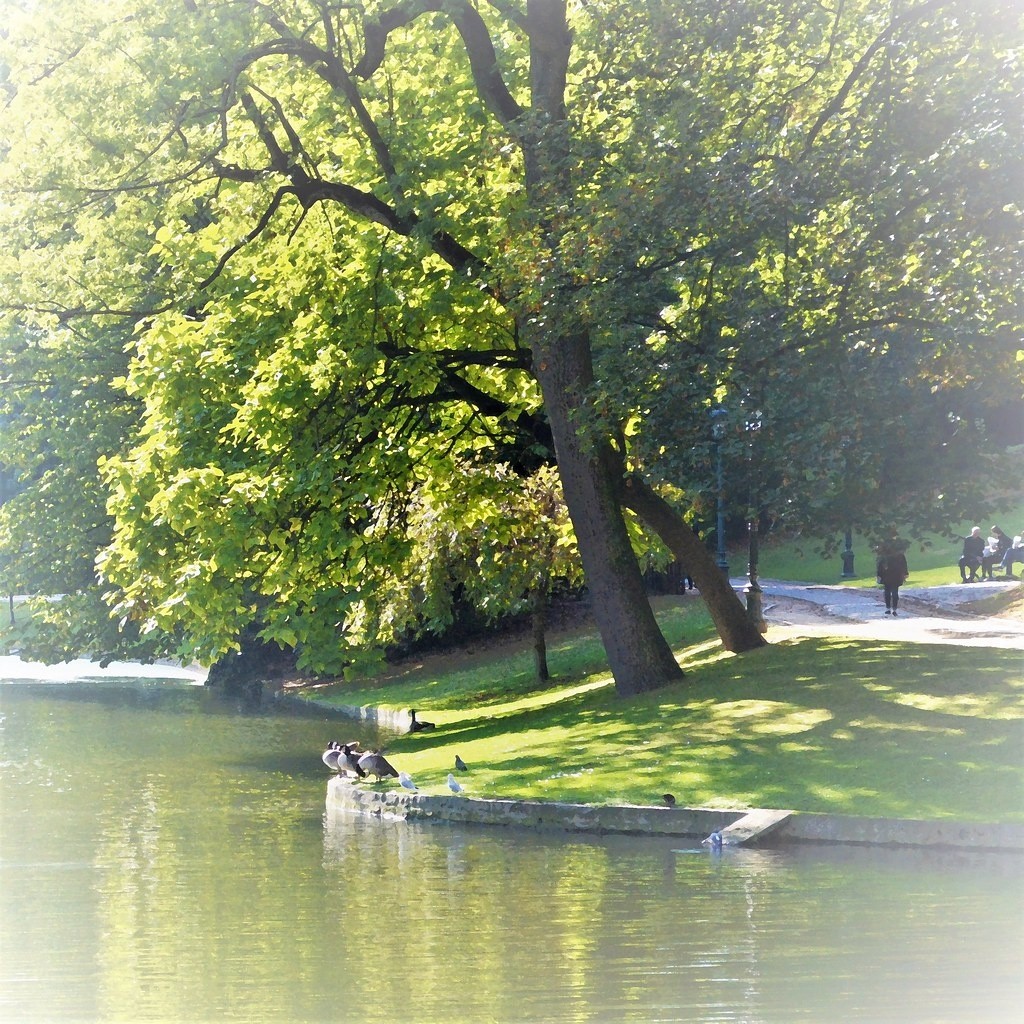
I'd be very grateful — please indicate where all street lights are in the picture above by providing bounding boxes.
[709,404,732,585]
[738,387,768,635]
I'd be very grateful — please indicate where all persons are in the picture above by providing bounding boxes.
[992,530,1024,580]
[979,525,1013,581]
[958,526,985,583]
[877,540,909,616]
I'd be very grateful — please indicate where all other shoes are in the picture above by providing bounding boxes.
[993,566,1002,572]
[892,611,898,618]
[884,611,891,617]
[962,575,1000,583]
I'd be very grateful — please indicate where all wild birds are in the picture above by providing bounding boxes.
[660,793,675,807]
[455,755,467,773]
[710,828,722,846]
[447,773,463,794]
[407,709,435,733]
[323,740,400,785]
[398,771,419,792]
